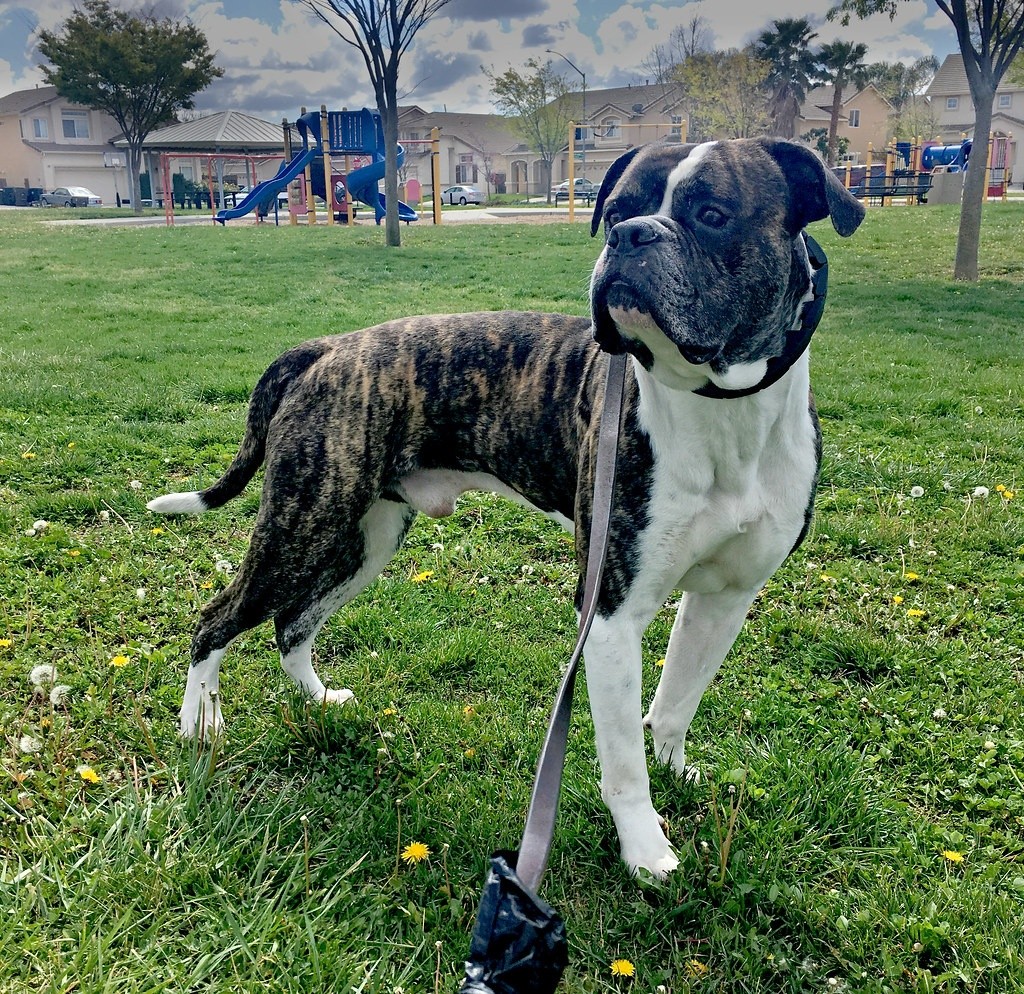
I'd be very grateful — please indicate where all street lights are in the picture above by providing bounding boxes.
[544,48,586,204]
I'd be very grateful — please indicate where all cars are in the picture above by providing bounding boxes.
[549,177,596,202]
[439,185,482,207]
[224,184,257,205]
[39,186,103,208]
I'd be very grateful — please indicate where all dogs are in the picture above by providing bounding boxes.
[143,136,864,878]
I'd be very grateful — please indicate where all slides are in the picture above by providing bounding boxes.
[346,142,419,221]
[214,149,317,221]
